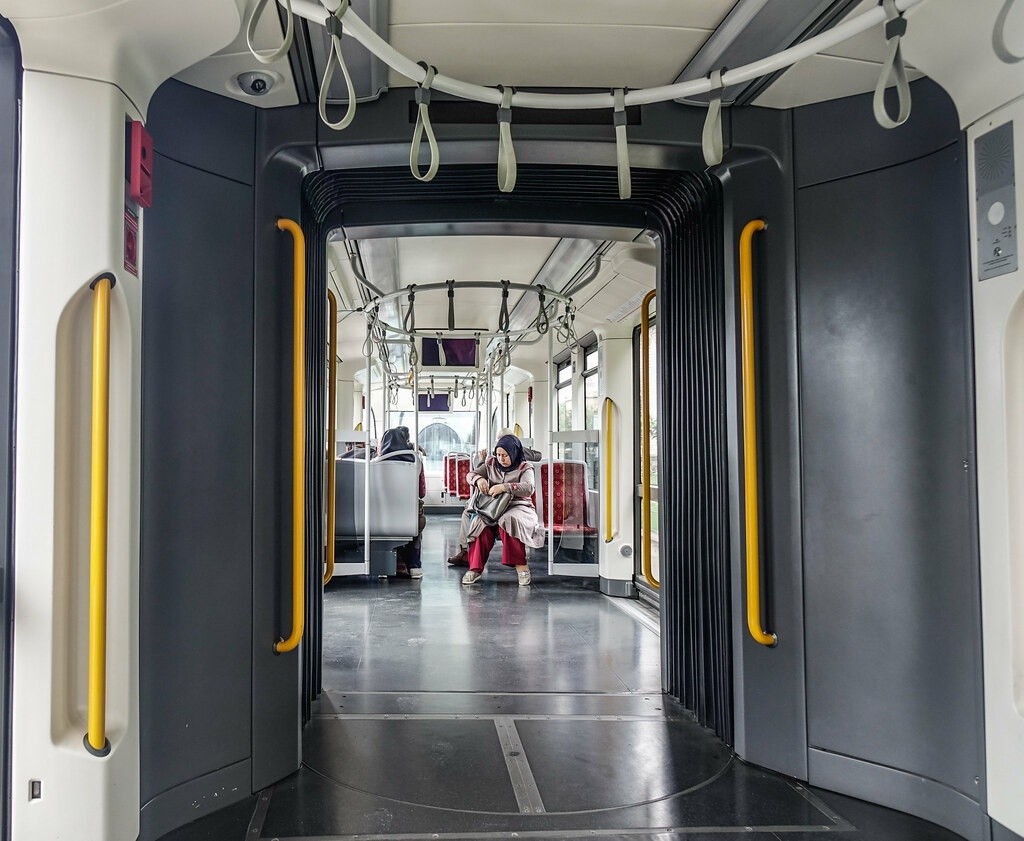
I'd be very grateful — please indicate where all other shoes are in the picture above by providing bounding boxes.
[516,565,531,586]
[462,571,481,584]
[447,548,468,566]
[409,567,423,579]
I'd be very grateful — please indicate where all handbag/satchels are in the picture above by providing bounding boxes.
[467,486,514,526]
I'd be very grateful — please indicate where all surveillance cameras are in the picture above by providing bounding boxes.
[238,73,274,96]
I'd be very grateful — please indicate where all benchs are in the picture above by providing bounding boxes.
[333,451,420,545]
[442,451,598,561]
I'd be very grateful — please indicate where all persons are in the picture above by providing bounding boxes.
[336,427,427,580]
[447,427,546,587]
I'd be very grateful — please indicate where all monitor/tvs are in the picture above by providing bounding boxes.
[414,334,486,372]
[418,391,454,414]
